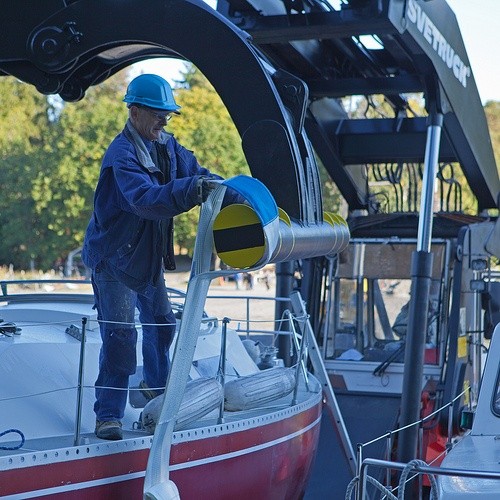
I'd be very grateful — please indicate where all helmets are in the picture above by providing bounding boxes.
[122,73,181,110]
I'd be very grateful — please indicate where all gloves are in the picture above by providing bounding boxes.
[196,176,220,206]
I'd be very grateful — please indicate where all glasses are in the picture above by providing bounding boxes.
[136,105,172,121]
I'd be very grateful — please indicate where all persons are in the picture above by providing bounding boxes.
[393,282,437,347]
[81,74,253,438]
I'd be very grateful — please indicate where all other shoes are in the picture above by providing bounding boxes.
[95,418,123,440]
[138,381,165,400]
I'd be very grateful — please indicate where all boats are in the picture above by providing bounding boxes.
[0,0,500,500]
